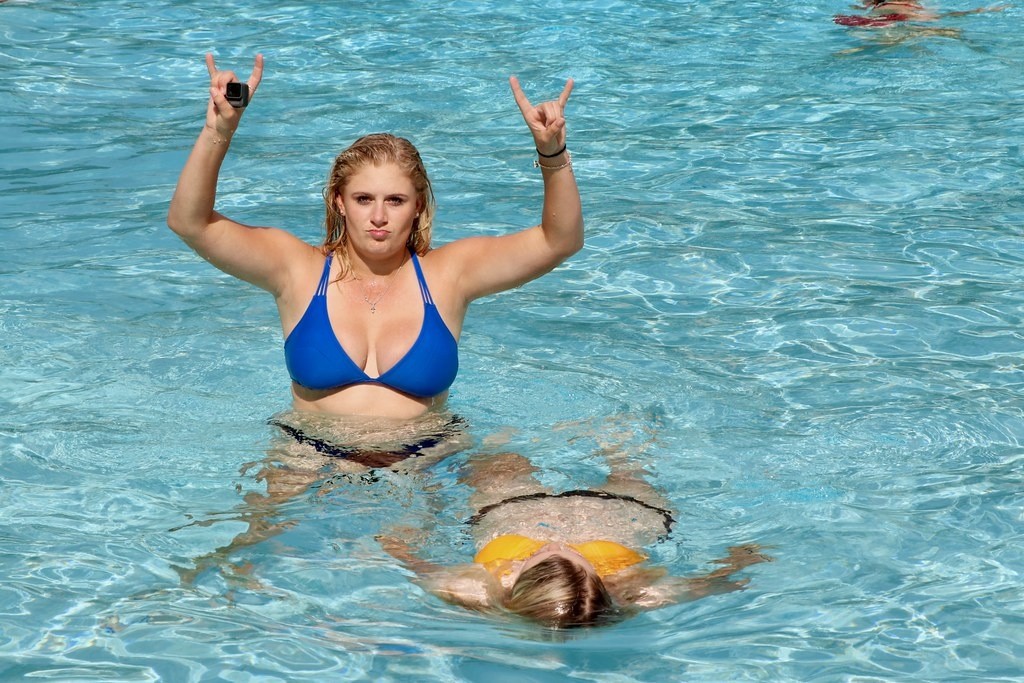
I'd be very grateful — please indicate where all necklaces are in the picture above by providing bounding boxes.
[348,247,408,313]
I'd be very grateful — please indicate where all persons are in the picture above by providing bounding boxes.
[833,0,1015,54]
[168,53,584,419]
[476,535,632,623]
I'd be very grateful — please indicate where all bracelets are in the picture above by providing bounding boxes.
[534,151,571,170]
[536,144,566,158]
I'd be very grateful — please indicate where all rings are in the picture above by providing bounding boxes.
[248,84,255,92]
